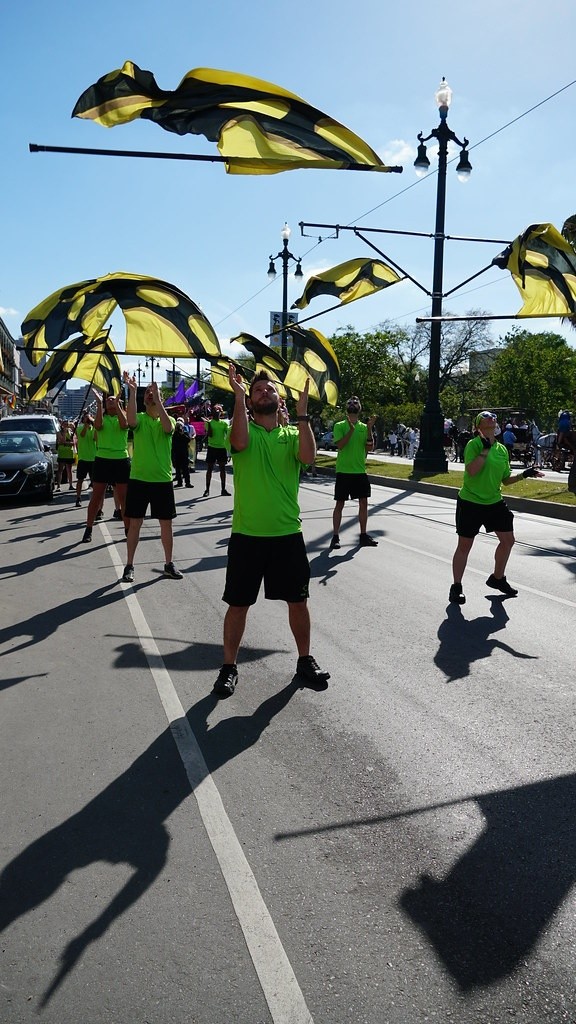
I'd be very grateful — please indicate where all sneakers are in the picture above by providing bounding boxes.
[212,666,239,698]
[163,562,184,579]
[122,566,136,582]
[296,654,330,683]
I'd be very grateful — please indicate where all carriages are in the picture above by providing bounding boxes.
[397,405,576,472]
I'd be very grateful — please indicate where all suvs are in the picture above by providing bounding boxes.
[1,413,62,475]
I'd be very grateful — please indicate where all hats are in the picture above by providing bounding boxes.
[506,424,513,429]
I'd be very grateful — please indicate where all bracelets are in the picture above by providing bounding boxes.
[298,415,309,421]
[481,453,487,458]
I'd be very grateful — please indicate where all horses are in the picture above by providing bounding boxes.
[528,421,560,467]
[448,423,474,462]
[397,424,415,457]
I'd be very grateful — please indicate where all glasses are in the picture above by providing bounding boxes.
[346,399,359,406]
[478,412,497,425]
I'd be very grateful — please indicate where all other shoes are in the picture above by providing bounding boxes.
[82,526,93,543]
[76,500,82,507]
[69,487,76,490]
[94,509,105,521]
[485,573,518,594]
[174,484,183,488]
[56,487,62,492]
[329,536,341,549]
[221,490,231,496]
[203,490,209,497]
[185,484,194,488]
[113,508,124,521]
[449,584,466,604]
[359,534,378,546]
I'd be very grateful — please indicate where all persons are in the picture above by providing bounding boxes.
[373,433,378,450]
[443,411,576,494]
[383,428,419,459]
[56,371,231,581]
[331,397,378,547]
[214,364,330,694]
[314,427,329,450]
[450,411,544,605]
[13,401,52,414]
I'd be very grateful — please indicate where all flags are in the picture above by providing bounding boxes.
[209,324,338,408]
[22,271,222,401]
[73,62,392,176]
[492,223,576,319]
[291,258,403,310]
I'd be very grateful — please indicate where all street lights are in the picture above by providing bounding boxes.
[267,220,305,366]
[412,74,473,479]
[132,361,146,387]
[145,355,162,384]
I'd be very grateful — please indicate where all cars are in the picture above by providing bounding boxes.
[316,432,338,451]
[0,431,56,500]
